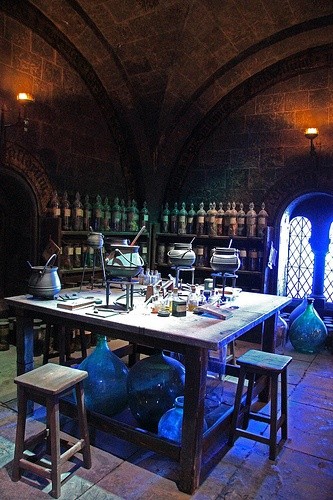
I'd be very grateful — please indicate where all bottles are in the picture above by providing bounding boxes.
[141,242,175,264]
[0,316,96,356]
[126,342,186,432]
[235,248,264,271]
[62,243,105,270]
[275,294,333,354]
[71,332,130,418]
[138,268,222,318]
[158,395,208,445]
[47,190,150,233]
[194,244,216,268]
[159,201,269,237]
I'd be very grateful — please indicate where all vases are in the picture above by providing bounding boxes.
[158,396,208,445]
[127,349,186,433]
[72,333,129,417]
[271,298,328,353]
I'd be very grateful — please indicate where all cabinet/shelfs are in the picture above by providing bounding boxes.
[43,217,274,344]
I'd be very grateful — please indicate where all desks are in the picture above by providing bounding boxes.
[4,279,291,496]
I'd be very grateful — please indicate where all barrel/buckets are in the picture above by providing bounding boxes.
[26,253,62,300]
[87,231,103,249]
[104,244,144,280]
[210,247,241,275]
[104,237,131,255]
[167,243,196,268]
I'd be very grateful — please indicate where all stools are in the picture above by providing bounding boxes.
[12,363,92,500]
[43,324,87,366]
[227,349,292,460]
[206,342,235,380]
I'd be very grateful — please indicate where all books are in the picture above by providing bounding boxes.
[57,298,95,311]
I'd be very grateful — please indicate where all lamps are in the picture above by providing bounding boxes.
[304,128,318,159]
[4,92,34,128]
